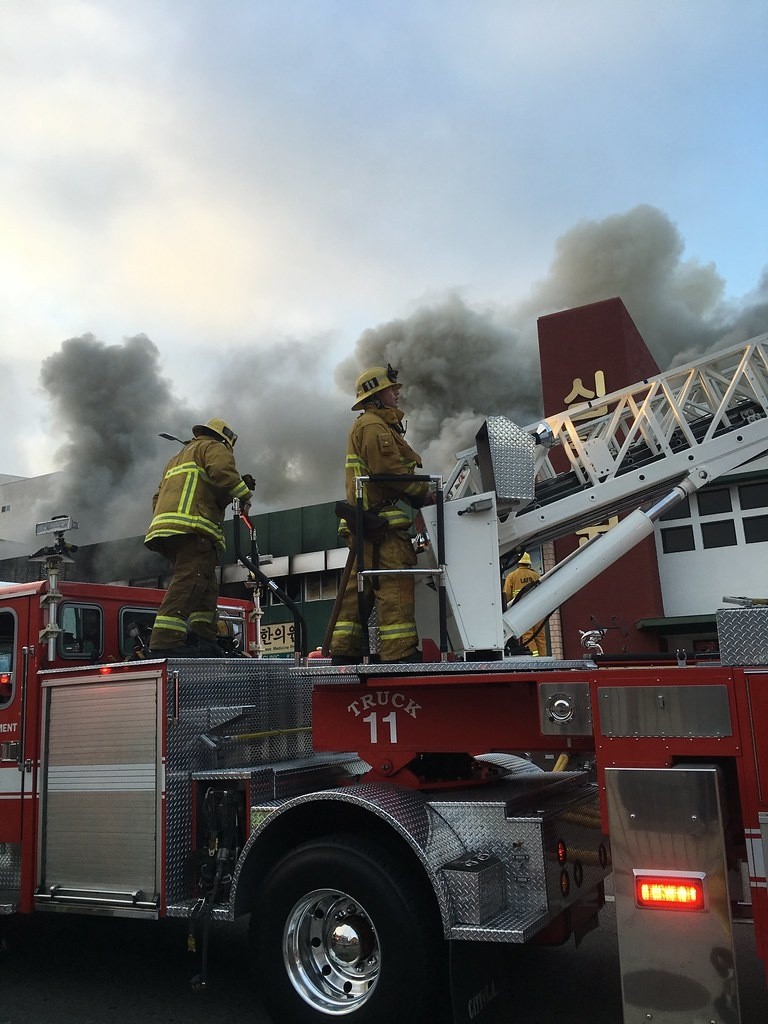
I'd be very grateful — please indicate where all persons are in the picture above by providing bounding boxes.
[330,367,437,665]
[503,552,547,657]
[143,417,253,658]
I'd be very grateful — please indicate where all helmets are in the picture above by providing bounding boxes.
[191,417,239,454]
[350,363,404,411]
[517,551,533,565]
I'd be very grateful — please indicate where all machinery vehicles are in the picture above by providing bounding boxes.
[1,329,768,1017]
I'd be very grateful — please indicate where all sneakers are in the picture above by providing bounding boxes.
[331,653,364,665]
[148,642,201,658]
[381,650,422,664]
[189,623,250,658]
